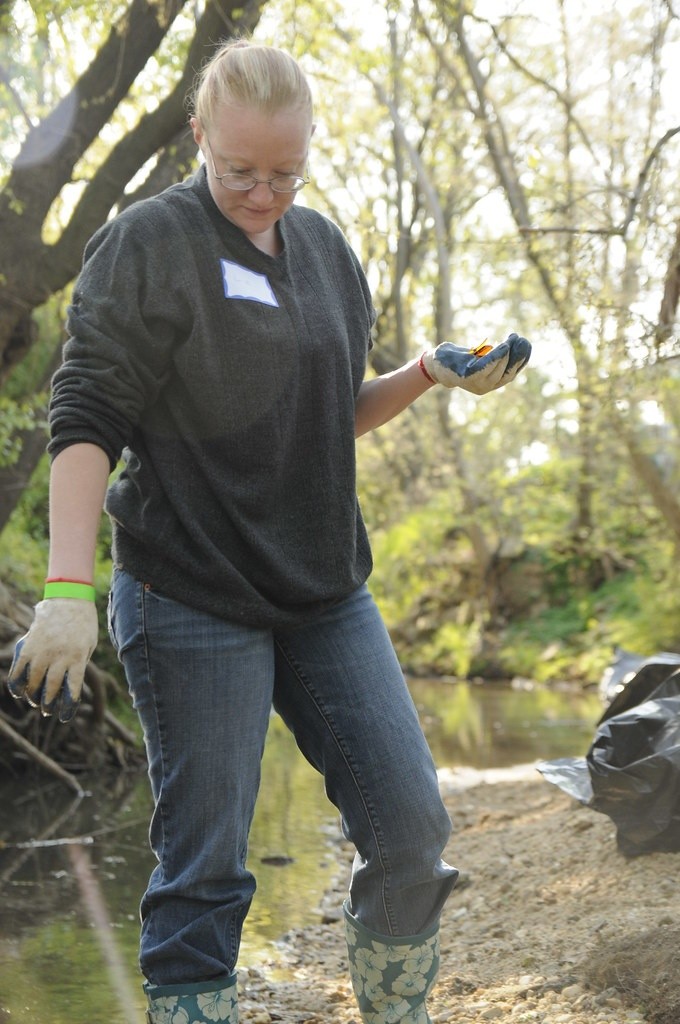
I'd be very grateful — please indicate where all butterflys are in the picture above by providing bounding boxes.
[470,336,493,356]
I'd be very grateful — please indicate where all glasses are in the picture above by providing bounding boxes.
[200,121,312,193]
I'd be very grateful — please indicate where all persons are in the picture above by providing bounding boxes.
[8,42,531,1023]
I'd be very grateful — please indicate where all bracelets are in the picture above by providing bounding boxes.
[43,578,96,603]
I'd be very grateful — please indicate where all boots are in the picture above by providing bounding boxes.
[140,971,238,1024]
[342,900,441,1024]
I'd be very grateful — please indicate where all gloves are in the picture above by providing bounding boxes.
[419,333,533,392]
[10,599,98,722]
